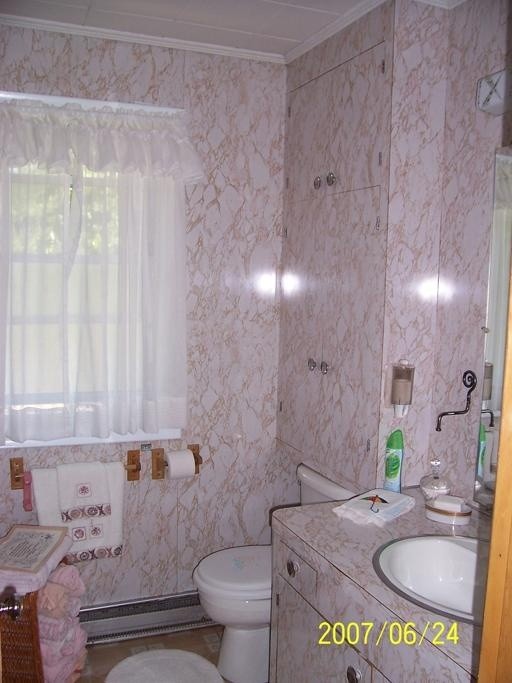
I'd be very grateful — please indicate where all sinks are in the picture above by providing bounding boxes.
[379,537,477,619]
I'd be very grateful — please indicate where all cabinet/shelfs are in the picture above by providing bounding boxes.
[269,487,477,683]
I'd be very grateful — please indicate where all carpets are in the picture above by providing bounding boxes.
[104,649,225,683]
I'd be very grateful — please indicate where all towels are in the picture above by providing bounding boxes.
[332,489,416,528]
[37,567,87,683]
[31,462,125,564]
[57,462,112,539]
[1,536,73,596]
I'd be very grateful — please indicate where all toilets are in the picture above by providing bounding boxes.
[191,463,356,683]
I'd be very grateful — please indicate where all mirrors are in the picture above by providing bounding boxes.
[474,147,512,512]
